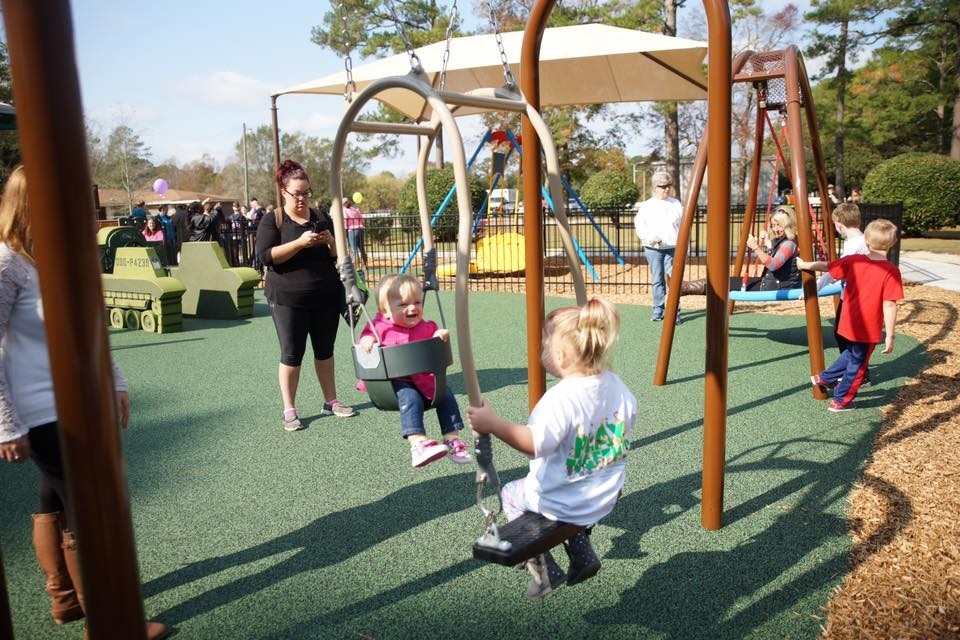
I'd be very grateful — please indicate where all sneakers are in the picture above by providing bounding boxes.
[444,438,474,464]
[322,401,356,417]
[410,441,448,468]
[282,411,304,431]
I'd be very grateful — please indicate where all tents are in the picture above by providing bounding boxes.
[270,23,709,208]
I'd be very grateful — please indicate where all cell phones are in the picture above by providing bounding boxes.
[315,219,329,235]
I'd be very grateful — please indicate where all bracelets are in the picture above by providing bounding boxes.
[753,246,759,251]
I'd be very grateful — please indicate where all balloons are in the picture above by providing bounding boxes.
[353,193,362,203]
[153,179,168,197]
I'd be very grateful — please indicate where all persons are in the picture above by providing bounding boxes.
[0,165,166,639]
[795,219,903,412]
[132,199,225,264]
[780,189,796,208]
[667,206,802,298]
[255,161,356,430]
[800,203,872,389]
[315,201,325,212]
[355,274,473,469]
[467,298,637,601]
[847,187,861,203]
[343,200,368,272]
[634,172,684,325]
[229,201,248,267]
[240,198,273,267]
[828,184,839,208]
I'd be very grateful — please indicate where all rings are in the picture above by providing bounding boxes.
[15,456,21,460]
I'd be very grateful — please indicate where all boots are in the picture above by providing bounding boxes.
[527,554,567,599]
[566,533,601,587]
[60,528,167,640]
[32,512,85,625]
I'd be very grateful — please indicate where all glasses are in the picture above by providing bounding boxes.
[284,187,313,201]
[656,183,673,189]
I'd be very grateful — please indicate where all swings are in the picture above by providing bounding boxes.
[731,90,842,303]
[322,4,625,571]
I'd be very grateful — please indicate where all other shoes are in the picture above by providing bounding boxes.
[828,399,856,413]
[811,375,839,387]
[651,311,664,321]
[675,315,682,324]
